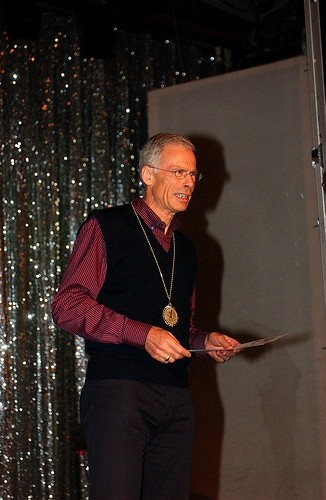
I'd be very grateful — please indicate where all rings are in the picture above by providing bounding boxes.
[164,355,171,363]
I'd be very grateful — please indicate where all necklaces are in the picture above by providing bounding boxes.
[130,201,179,327]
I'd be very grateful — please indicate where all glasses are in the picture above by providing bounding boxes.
[150,166,202,182]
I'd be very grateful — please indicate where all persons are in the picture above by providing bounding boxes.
[50,133,243,500]
